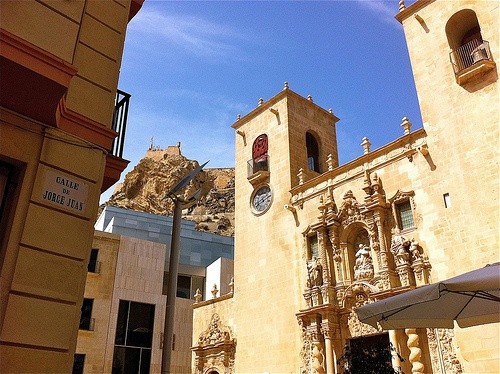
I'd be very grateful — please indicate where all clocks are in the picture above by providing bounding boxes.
[249,183,274,217]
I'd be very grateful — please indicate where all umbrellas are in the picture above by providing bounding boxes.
[354,261,499,331]
